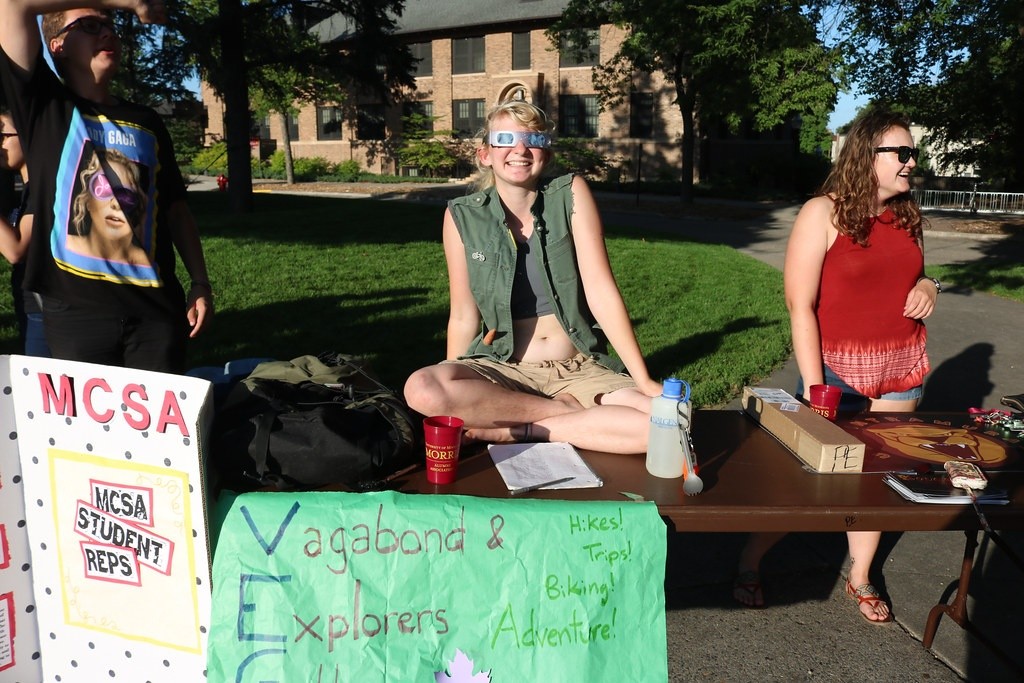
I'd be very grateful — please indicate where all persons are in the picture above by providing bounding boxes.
[403,96,686,454]
[0,0,214,376]
[0,104,35,355]
[734,110,942,626]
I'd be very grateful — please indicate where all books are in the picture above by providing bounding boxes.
[881,472,1011,505]
[487,441,604,490]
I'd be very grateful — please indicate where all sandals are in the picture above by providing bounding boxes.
[736,549,767,609]
[844,578,894,627]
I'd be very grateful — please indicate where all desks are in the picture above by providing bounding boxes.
[252,409,1024,683]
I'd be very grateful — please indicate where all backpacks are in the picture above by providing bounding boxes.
[205,350,413,496]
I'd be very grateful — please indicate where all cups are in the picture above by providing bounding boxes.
[424,416,463,484]
[809,384,843,422]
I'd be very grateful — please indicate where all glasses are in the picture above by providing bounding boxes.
[50,17,122,38]
[483,131,553,149]
[875,144,921,164]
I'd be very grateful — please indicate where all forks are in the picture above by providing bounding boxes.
[677,420,703,496]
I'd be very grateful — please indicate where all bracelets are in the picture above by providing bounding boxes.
[191,280,211,292]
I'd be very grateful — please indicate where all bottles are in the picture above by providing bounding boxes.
[645,377,694,479]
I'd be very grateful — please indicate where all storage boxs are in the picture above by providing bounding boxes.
[742,386,866,473]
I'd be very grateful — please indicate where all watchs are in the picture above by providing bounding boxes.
[918,277,942,294]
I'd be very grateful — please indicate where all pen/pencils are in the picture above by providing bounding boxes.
[510,476,575,495]
